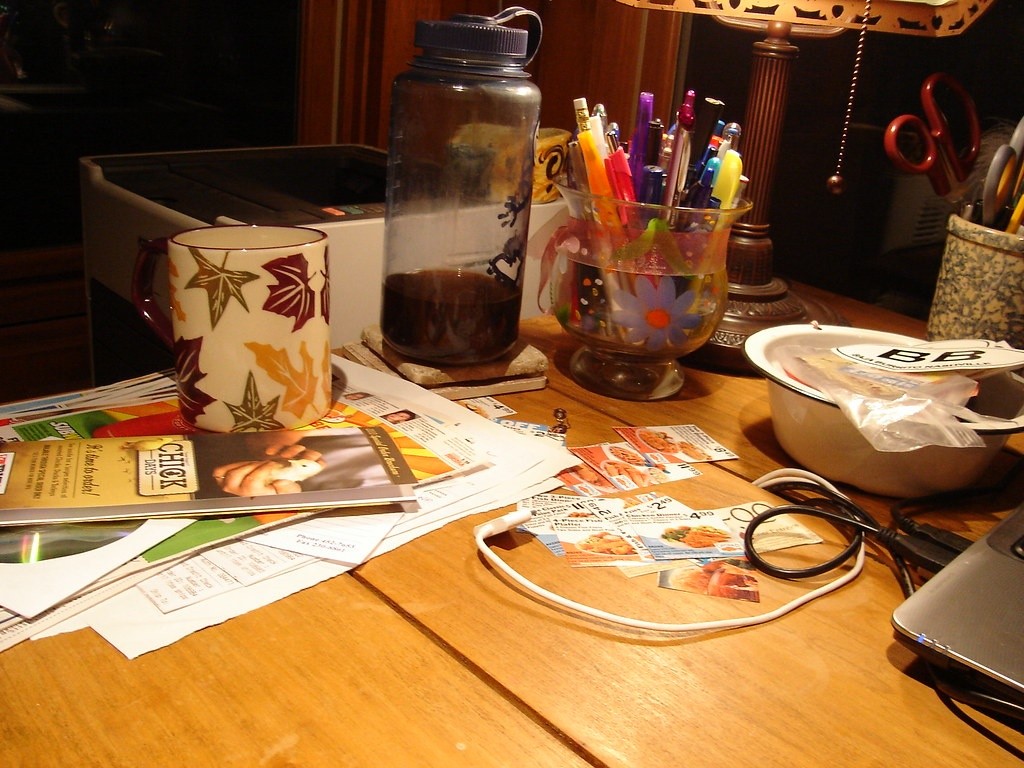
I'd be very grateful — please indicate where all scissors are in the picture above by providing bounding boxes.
[885,69,982,206]
[981,117,1024,231]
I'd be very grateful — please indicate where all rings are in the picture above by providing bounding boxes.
[212,474,226,480]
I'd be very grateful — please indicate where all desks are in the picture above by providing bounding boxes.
[0,279,1024,768]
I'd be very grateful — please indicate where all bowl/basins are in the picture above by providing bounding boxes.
[745,322,1024,495]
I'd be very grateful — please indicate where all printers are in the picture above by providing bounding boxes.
[81,143,570,350]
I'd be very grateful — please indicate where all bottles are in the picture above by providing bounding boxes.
[378,6,543,363]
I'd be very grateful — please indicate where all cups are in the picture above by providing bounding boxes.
[550,172,754,402]
[166,225,334,433]
[927,214,1024,350]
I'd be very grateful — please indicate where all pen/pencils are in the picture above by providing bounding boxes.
[557,90,750,346]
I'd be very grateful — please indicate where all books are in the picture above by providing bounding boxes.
[0,426,420,531]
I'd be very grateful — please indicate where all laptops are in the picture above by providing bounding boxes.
[890,500,1023,721]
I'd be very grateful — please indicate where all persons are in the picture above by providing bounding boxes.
[211,428,391,498]
[342,392,371,401]
[382,409,416,425]
